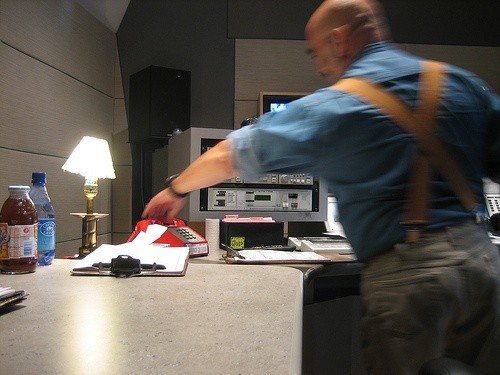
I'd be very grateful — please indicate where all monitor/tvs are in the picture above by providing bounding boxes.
[258,91,317,118]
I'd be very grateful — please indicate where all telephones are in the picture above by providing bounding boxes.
[127,217,209,257]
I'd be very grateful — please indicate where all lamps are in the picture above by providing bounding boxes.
[62,136,116,256]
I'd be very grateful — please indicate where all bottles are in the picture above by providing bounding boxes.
[0,186,39,275]
[27,171,56,266]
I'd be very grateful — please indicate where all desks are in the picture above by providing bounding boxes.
[0,249,364,375]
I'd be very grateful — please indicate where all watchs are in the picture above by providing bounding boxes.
[166,174,190,198]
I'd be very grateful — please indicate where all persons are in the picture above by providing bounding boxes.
[140,0,500,375]
